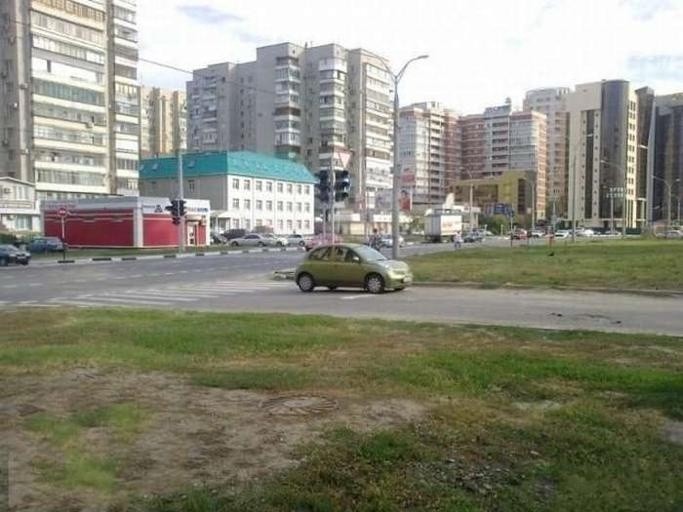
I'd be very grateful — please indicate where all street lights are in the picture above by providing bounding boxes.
[651,176,680,226]
[362,49,428,259]
[459,166,472,216]
[601,160,626,235]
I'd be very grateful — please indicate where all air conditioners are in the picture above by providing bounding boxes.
[1,24,94,194]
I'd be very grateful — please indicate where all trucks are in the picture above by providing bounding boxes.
[424,215,473,243]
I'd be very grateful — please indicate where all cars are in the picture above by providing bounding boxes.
[512,227,621,240]
[668,230,682,239]
[464,232,483,242]
[0,244,31,266]
[382,235,405,248]
[214,233,341,252]
[295,243,411,293]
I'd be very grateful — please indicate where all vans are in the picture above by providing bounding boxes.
[32,237,64,252]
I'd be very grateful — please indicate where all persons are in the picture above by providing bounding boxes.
[548,226,555,249]
[452,229,463,250]
[397,188,411,212]
[367,227,382,253]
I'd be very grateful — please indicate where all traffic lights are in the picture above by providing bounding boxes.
[335,170,350,201]
[172,200,186,224]
[315,170,330,203]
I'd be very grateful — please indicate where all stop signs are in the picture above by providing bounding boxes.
[59,208,66,216]
